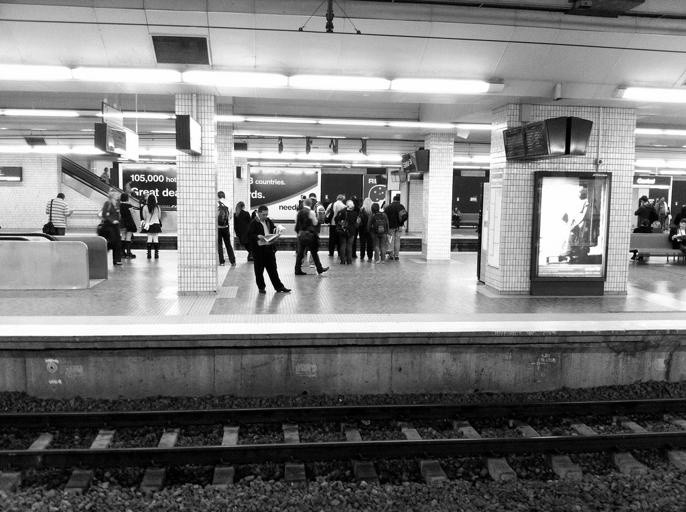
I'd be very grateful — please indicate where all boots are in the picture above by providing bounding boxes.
[146,242,159,259]
[121,240,135,258]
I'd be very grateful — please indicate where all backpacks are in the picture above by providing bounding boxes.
[372,213,387,236]
[314,203,326,224]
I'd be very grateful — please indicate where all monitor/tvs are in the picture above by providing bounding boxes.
[93,123,128,154]
[502,115,593,162]
[148,35,214,71]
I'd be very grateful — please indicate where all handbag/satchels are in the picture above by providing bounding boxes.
[336,220,352,238]
[399,209,407,224]
[324,207,335,224]
[43,221,57,235]
[218,200,229,227]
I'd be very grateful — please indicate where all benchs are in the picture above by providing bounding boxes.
[631,232,685,264]
[458,213,479,228]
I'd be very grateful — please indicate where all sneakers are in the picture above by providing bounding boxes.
[114,261,122,265]
[310,264,315,267]
[220,261,237,267]
[328,252,399,265]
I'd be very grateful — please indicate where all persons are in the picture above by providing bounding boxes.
[101,167,110,184]
[217,191,236,266]
[46,193,73,235]
[246,206,291,293]
[98,179,162,265]
[234,201,254,261]
[631,195,686,259]
[295,193,408,275]
[452,206,462,228]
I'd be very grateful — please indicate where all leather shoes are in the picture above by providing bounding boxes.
[318,267,329,275]
[258,288,267,295]
[276,287,291,293]
[295,271,307,276]
